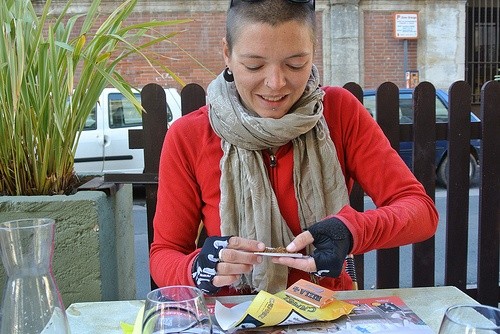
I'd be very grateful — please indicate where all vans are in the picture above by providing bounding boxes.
[33,86,182,176]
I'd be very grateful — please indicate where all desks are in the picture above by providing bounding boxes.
[41,285,500,334]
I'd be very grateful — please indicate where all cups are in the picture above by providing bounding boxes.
[0,217,73,334]
[438,305,500,334]
[141,285,213,334]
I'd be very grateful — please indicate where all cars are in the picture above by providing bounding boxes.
[362,88,481,186]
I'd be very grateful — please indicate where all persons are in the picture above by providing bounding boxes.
[149,0,439,299]
